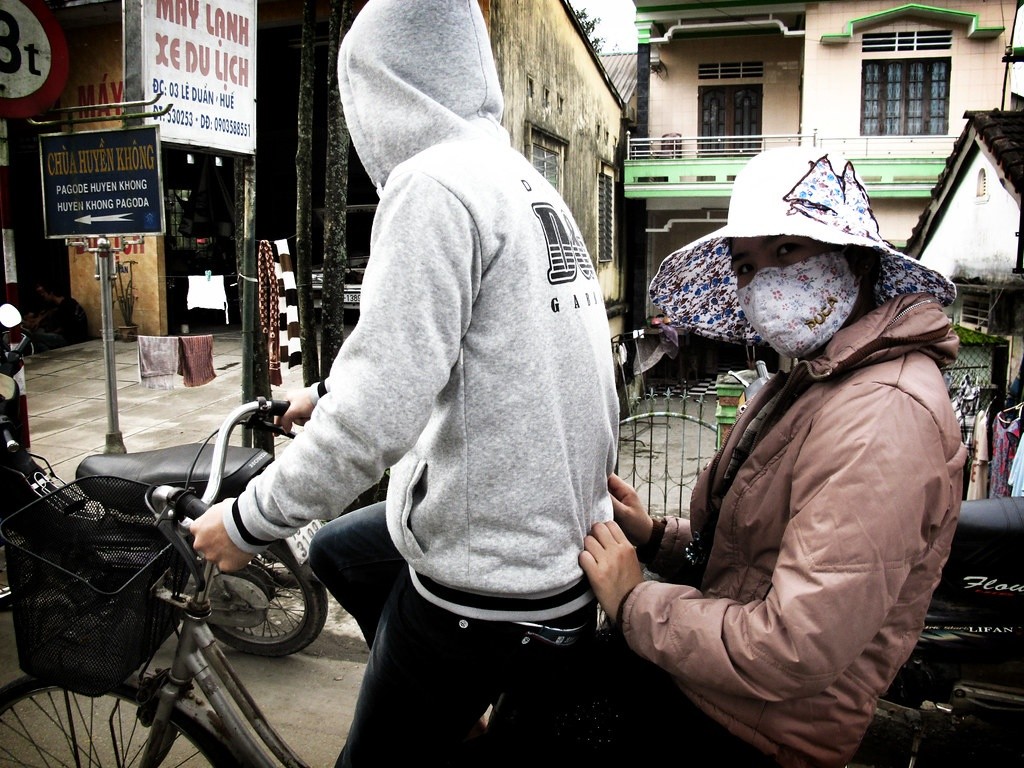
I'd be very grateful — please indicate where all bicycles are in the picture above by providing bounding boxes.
[0,394,313,768]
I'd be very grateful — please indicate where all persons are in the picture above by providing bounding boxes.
[22,274,88,349]
[576,146,967,768]
[191,0,620,768]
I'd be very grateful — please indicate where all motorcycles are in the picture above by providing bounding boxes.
[0,337,328,658]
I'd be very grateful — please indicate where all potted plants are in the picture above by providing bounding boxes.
[111,260,139,342]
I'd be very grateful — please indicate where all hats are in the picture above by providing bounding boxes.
[649,145,957,346]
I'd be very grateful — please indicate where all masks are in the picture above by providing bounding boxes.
[735,250,860,358]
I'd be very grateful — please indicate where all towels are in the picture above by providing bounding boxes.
[177,333,218,388]
[137,334,179,392]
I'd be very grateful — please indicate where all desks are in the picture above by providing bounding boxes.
[640,326,697,396]
[715,400,745,451]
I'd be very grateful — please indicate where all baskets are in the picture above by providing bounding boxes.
[0,475,198,698]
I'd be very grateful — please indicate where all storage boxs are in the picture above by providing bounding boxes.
[715,375,744,406]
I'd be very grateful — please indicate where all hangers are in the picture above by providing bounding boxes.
[998,402,1023,423]
